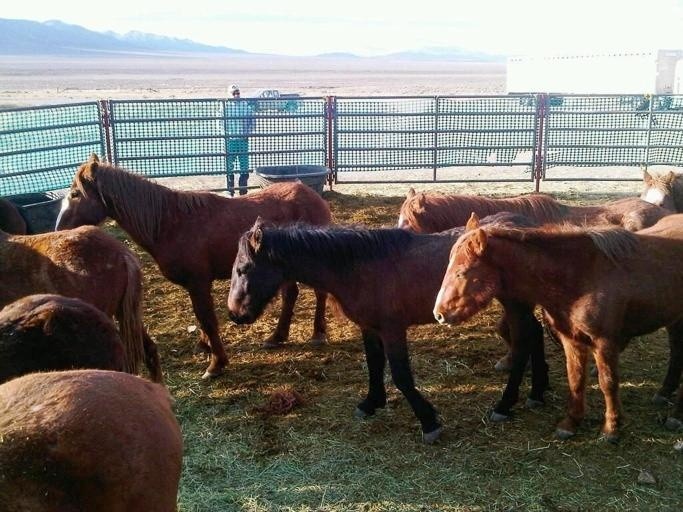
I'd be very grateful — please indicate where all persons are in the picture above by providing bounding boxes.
[215,83,256,198]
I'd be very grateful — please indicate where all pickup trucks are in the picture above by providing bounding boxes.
[250,89,303,113]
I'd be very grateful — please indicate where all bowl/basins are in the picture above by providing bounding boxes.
[255,166,331,201]
[3,188,65,235]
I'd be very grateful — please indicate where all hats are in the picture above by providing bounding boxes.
[230,83,239,93]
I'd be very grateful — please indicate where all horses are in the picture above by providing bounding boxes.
[0,195,34,235]
[0,225,163,385]
[397,187,683,377]
[0,368,184,512]
[54,152,332,380]
[226,210,549,445]
[639,169,683,214]
[432,210,683,446]
[0,294,126,385]
